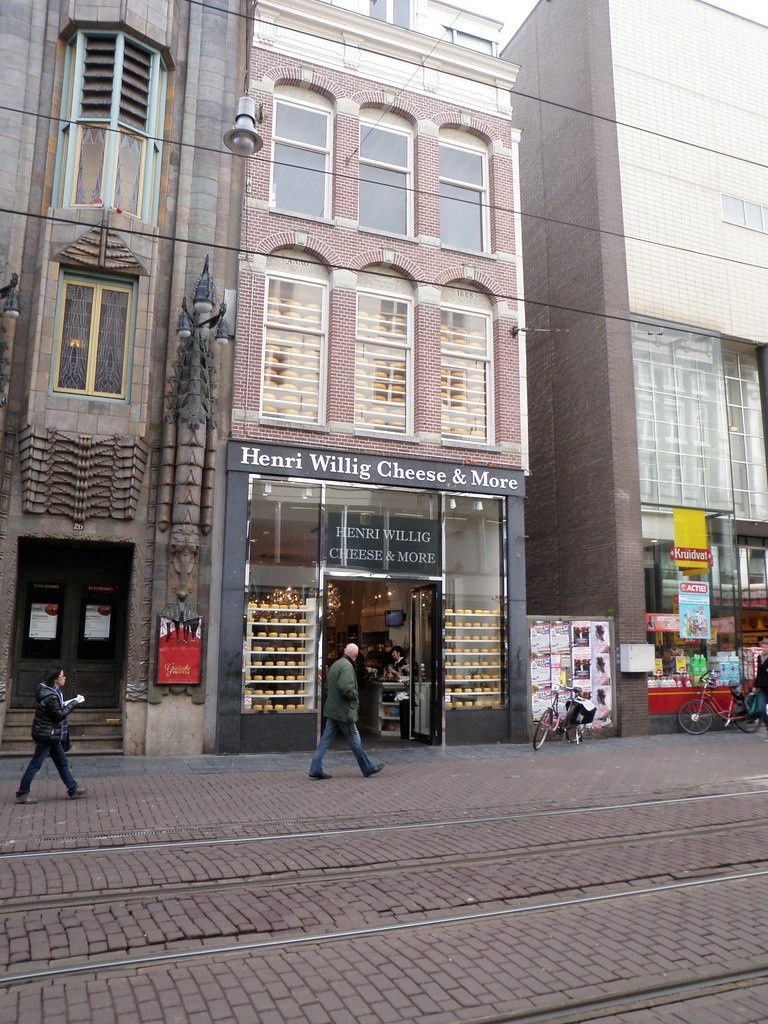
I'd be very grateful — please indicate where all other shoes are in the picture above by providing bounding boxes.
[370,763,385,775]
[309,772,332,779]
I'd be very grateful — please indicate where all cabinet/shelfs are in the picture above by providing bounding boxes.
[258,300,329,420]
[380,701,400,721]
[441,329,491,439]
[241,598,315,713]
[355,315,409,435]
[444,614,506,710]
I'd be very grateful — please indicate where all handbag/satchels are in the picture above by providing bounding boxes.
[567,696,597,723]
[744,693,765,717]
[62,730,72,751]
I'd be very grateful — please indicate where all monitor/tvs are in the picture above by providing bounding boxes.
[384,610,404,626]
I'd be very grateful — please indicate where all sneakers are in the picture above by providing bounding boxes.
[17,791,38,804]
[70,785,89,800]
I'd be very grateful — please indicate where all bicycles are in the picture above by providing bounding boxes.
[677,669,763,735]
[533,683,597,751]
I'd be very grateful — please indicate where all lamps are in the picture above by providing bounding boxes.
[478,499,484,512]
[264,484,272,492]
[0,272,23,320]
[224,96,265,157]
[176,252,230,346]
[449,497,457,510]
[71,339,80,365]
[307,487,312,497]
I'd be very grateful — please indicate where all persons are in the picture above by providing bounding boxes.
[595,657,609,685]
[646,616,656,629]
[357,644,368,672]
[15,666,89,803]
[576,627,585,638]
[752,639,768,742]
[387,646,407,677]
[377,640,395,668]
[596,688,611,717]
[577,659,587,671]
[309,643,385,779]
[595,625,610,653]
